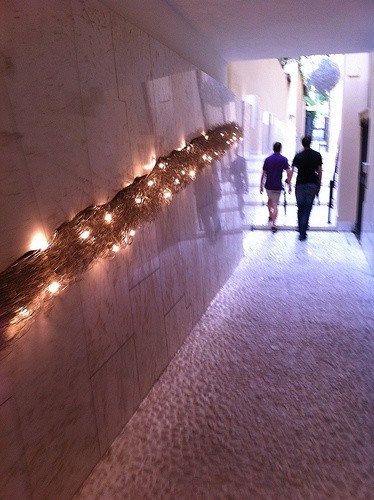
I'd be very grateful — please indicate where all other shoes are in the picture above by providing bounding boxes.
[269,216,272,222]
[272,226,277,232]
[299,233,306,241]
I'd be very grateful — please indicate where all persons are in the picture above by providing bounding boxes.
[285,135,323,241]
[195,146,250,243]
[259,141,291,233]
